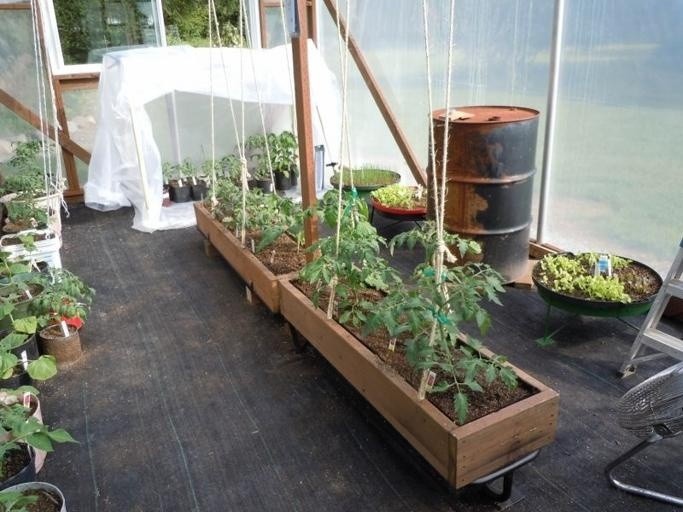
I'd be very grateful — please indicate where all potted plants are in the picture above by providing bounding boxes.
[528,247,664,343]
[156,127,564,501]
[0,135,97,512]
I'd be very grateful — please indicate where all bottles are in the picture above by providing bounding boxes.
[597,247,612,279]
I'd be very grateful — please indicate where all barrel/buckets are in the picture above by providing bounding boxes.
[426,105,539,285]
[0,191,62,249]
[0,230,62,284]
